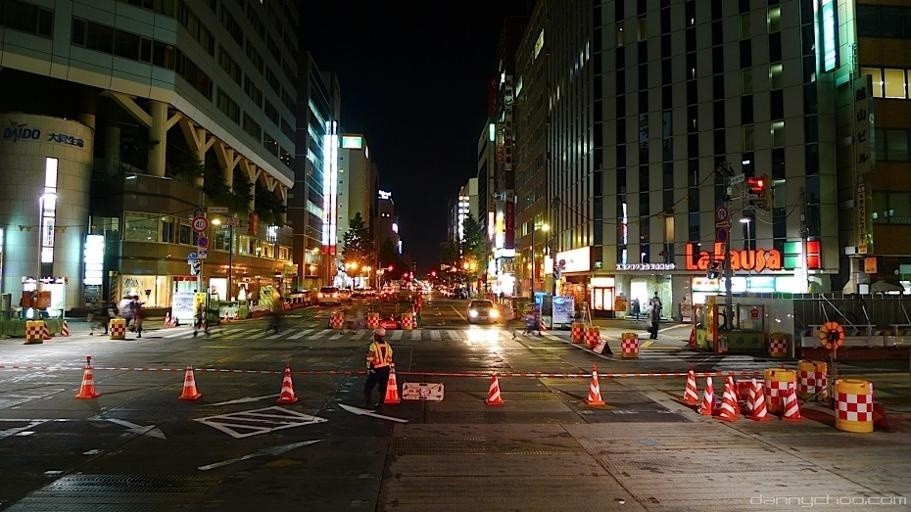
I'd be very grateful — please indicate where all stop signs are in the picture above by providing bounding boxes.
[197,237,209,247]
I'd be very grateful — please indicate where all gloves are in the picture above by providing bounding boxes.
[366,369,370,376]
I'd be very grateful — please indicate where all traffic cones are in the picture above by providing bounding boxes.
[277,362,298,403]
[178,364,201,400]
[76,366,101,398]
[412,313,417,329]
[485,373,503,404]
[61,321,71,336]
[224,312,229,322]
[584,366,604,405]
[539,318,547,330]
[43,323,50,338]
[679,372,804,422]
[384,364,400,404]
[163,311,170,326]
[686,328,696,348]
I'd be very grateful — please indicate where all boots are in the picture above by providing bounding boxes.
[376,392,385,412]
[358,390,370,409]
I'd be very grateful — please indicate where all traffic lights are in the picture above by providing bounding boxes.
[189,260,200,276]
[707,261,719,279]
[248,212,257,235]
[748,172,769,196]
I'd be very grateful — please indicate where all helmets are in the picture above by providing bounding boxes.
[373,327,385,337]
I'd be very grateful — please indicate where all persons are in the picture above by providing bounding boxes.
[246,290,253,306]
[266,297,284,335]
[359,325,394,407]
[632,297,641,320]
[653,291,661,304]
[85,294,143,338]
[650,304,661,340]
[192,302,209,336]
[522,312,543,336]
[412,291,424,327]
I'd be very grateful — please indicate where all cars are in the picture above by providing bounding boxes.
[450,288,465,300]
[318,286,375,305]
[467,299,497,325]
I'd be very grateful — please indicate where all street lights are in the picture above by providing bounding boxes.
[530,219,550,303]
[378,263,392,293]
[35,192,58,281]
[210,219,232,299]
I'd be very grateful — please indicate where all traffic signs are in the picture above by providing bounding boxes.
[191,215,208,234]
